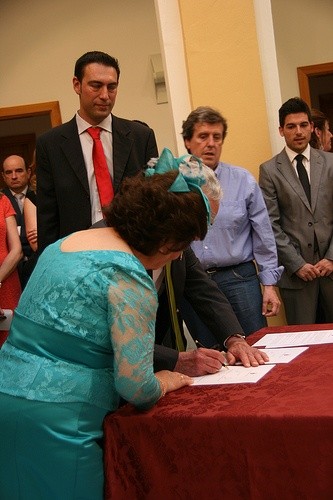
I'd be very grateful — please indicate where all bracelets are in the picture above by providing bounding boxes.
[155,374,165,397]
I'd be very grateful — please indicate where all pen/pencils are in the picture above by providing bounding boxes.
[194,340,232,372]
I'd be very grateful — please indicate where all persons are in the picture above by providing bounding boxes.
[36,51,160,257]
[178,106,284,354]
[85,160,270,409]
[0,191,23,347]
[25,151,38,279]
[256,97,333,325]
[308,110,333,151]
[1,148,211,500]
[1,155,40,293]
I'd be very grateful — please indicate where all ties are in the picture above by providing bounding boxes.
[163,260,183,353]
[16,194,25,216]
[295,155,312,207]
[85,127,115,221]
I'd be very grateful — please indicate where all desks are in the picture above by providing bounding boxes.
[104,324,332,500]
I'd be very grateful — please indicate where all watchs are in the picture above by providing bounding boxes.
[225,333,245,346]
